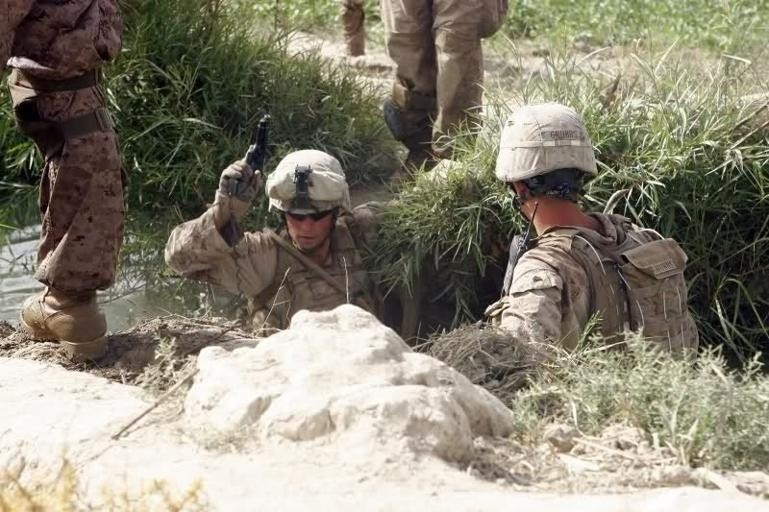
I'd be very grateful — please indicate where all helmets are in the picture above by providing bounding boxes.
[264,148,354,216]
[494,102,598,182]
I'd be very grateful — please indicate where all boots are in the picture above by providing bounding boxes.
[20,285,108,363]
[389,148,430,192]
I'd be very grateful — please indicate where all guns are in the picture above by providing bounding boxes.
[223,114,270,200]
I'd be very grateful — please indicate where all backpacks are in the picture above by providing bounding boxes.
[576,213,699,368]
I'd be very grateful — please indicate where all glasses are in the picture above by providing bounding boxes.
[289,210,330,221]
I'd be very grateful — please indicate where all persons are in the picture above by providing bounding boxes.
[162,149,418,338]
[485,102,700,365]
[341,0,508,183]
[0,0,124,361]
